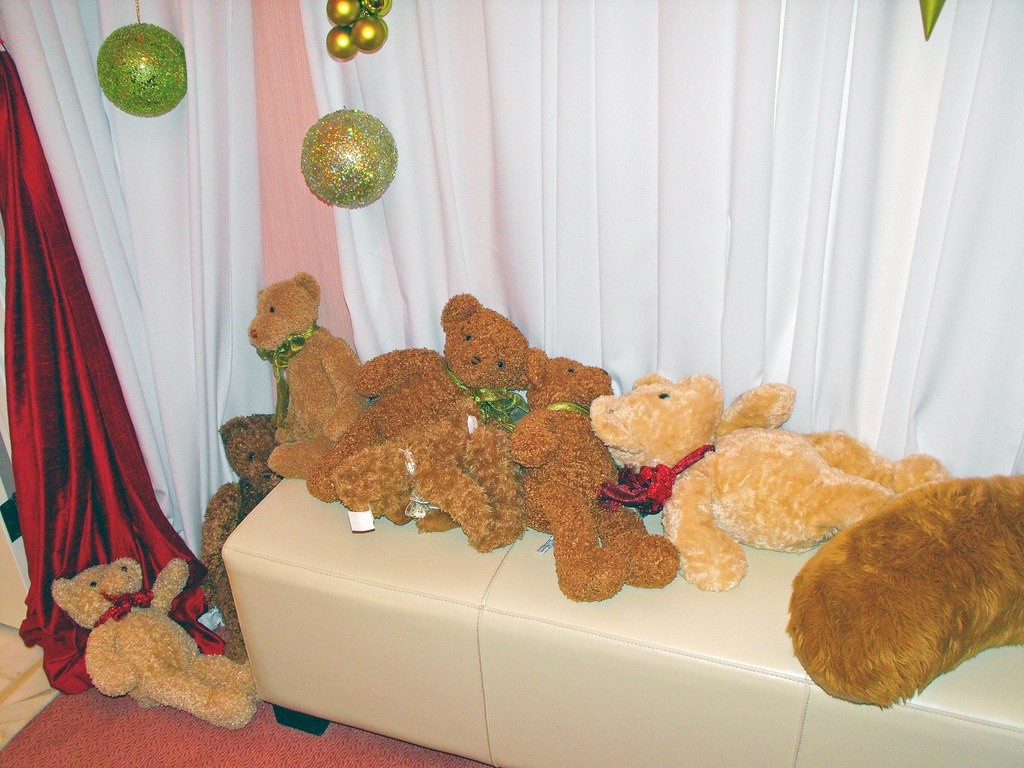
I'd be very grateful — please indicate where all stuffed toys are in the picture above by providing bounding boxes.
[588,373,951,591]
[331,397,527,552]
[50,557,261,730]
[511,349,681,603]
[200,415,284,664]
[788,473,1024,711]
[305,292,528,503]
[248,270,365,478]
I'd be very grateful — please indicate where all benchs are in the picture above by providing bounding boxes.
[223,479,1024,768]
